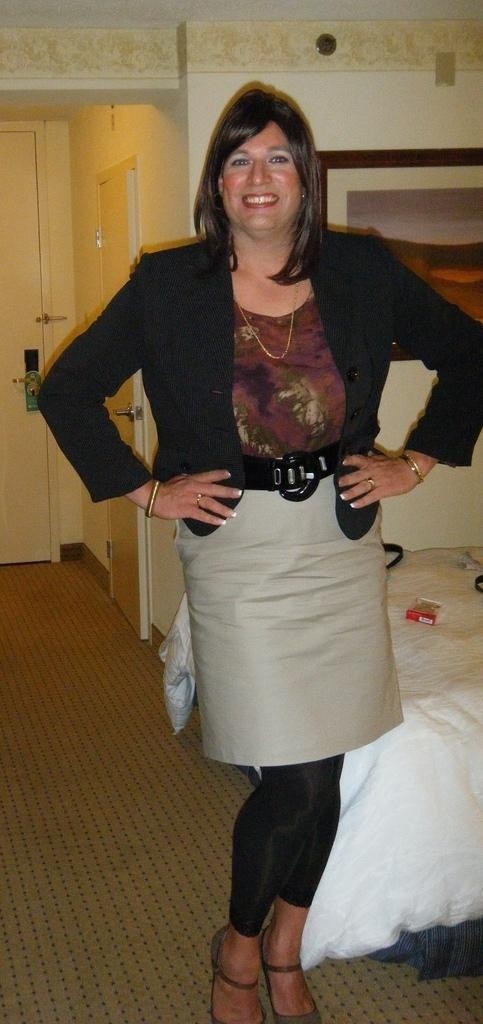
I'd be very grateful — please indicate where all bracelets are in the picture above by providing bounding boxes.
[145,479,163,517]
[401,449,426,486]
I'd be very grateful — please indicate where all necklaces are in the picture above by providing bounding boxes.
[231,262,297,360]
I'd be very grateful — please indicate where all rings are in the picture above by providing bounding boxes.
[366,477,374,489]
[196,494,201,504]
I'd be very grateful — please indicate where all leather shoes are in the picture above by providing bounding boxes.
[260,925,321,1024]
[210,926,266,1024]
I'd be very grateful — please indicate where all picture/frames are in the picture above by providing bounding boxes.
[313,147,483,361]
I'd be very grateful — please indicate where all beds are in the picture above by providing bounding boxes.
[253,544,482,970]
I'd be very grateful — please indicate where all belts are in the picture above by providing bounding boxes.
[244,441,337,491]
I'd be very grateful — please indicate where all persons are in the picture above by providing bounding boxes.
[34,78,480,1023]
